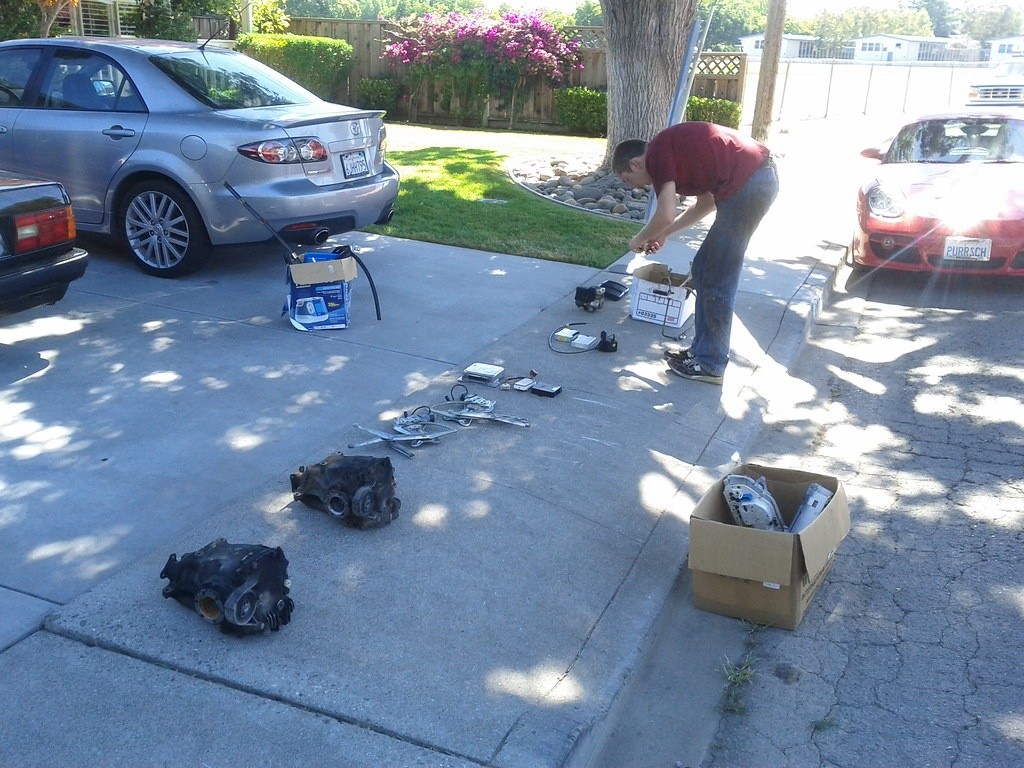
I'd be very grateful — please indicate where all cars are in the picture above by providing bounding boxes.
[0,34,405,279]
[0,175,90,313]
[850,107,1024,277]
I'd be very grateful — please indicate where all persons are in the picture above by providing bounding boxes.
[615,122,780,385]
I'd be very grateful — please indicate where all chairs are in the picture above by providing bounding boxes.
[62,73,209,112]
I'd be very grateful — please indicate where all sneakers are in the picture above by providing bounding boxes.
[664,346,691,359]
[667,358,724,384]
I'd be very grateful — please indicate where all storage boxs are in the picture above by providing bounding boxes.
[280,252,359,332]
[630,262,698,328]
[686,464,851,632]
